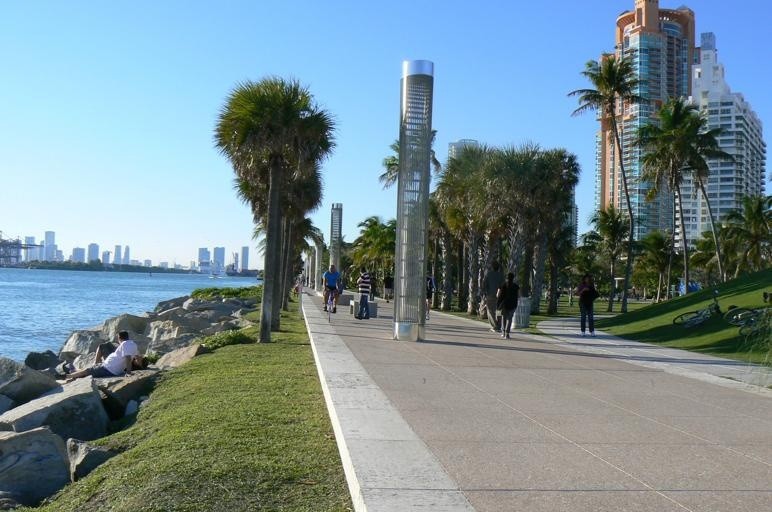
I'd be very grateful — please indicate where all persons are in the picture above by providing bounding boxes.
[426,271,436,320]
[322,264,342,313]
[578,278,599,337]
[64,330,136,378]
[369,273,378,301]
[355,267,371,319]
[383,271,394,302]
[93,341,147,370]
[496,272,520,338]
[481,260,505,333]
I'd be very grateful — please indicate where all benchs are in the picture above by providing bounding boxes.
[366,300,378,318]
[349,298,360,317]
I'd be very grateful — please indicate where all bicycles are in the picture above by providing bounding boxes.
[730,292,772,339]
[674,288,758,327]
[322,288,339,323]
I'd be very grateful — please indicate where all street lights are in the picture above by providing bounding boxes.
[305,232,323,293]
[327,203,344,274]
[391,58,434,344]
